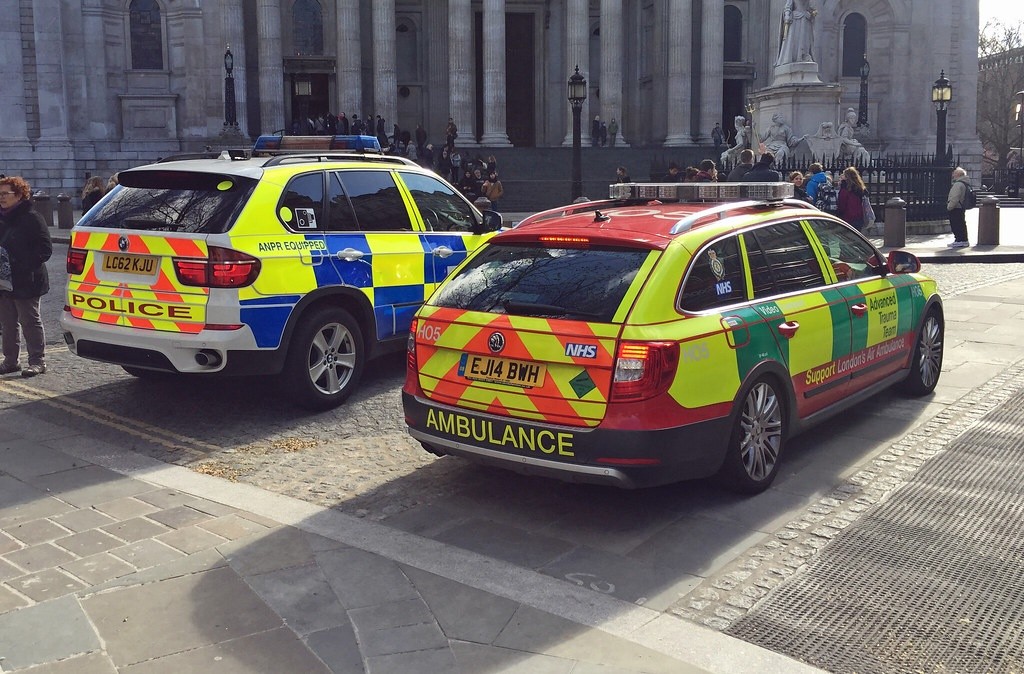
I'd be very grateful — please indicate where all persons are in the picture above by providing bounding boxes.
[711,123,725,151]
[591,116,618,147]
[617,167,631,183]
[721,116,746,169]
[662,159,717,183]
[727,150,779,182]
[82,172,119,216]
[839,113,874,167]
[758,114,809,173]
[789,163,866,233]
[0,176,52,375]
[947,168,969,247]
[774,0,818,65]
[292,112,504,211]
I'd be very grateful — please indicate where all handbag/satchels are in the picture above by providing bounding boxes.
[862,194,875,229]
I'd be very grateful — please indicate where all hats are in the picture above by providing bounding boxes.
[761,153,773,162]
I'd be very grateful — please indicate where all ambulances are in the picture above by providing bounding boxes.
[400,181,946,498]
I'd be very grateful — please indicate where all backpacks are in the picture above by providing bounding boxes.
[814,179,838,213]
[954,181,976,209]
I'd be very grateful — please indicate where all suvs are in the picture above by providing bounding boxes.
[61,135,511,415]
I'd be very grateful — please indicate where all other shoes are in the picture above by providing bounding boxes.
[22,364,46,375]
[0,359,21,374]
[948,242,969,247]
[453,182,455,185]
[456,182,458,185]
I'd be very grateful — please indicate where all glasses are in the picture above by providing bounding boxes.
[0,191,16,196]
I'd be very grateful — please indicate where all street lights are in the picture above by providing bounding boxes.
[567,65,587,204]
[932,69,951,202]
